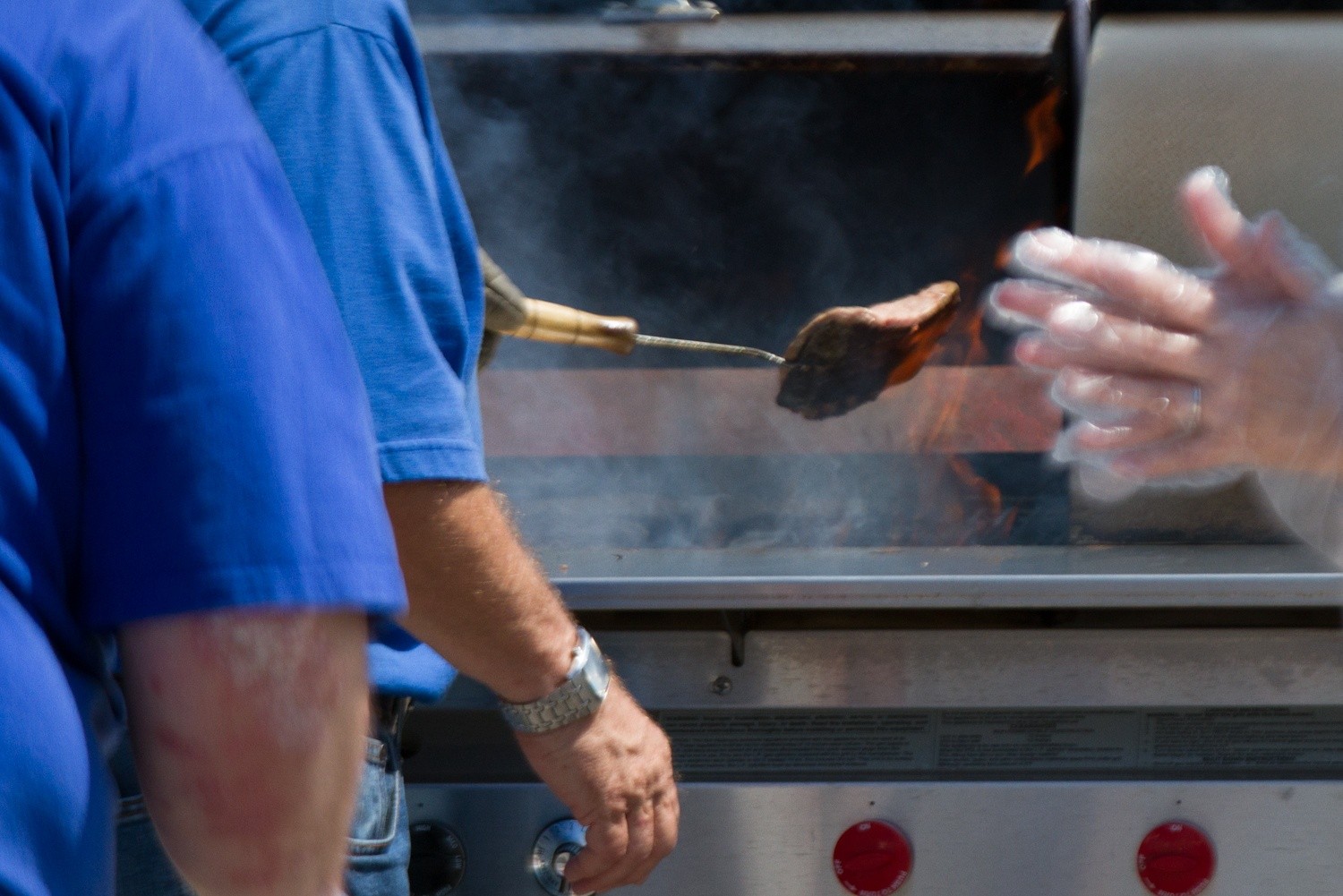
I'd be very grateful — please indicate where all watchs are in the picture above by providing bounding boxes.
[496,625,612,735]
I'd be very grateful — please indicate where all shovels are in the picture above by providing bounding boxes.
[501,280,962,419]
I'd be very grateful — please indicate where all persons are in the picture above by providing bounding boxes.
[185,0,685,896]
[987,164,1343,570]
[0,0,409,896]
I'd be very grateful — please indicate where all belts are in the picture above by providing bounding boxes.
[366,690,415,748]
